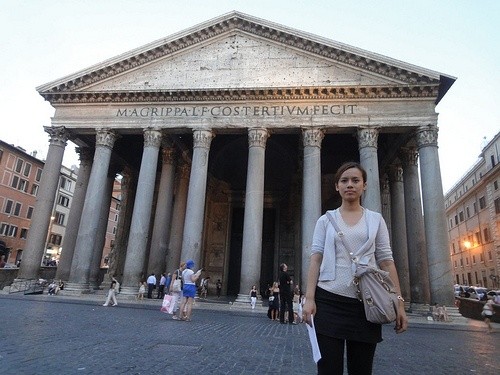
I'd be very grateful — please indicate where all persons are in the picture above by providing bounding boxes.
[198,274,210,299]
[455,288,500,333]
[262,280,306,325]
[432,303,448,321]
[179,261,201,321]
[216,279,222,297]
[301,161,407,375]
[103,272,120,306]
[41,279,64,295]
[135,272,172,300]
[280,263,297,325]
[168,262,187,319]
[250,285,258,310]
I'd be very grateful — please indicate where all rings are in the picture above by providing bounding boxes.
[302,314,305,316]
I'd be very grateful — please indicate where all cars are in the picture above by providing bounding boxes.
[454,285,500,300]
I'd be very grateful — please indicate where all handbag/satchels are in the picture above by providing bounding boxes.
[353,259,398,323]
[160,292,177,314]
[170,270,182,292]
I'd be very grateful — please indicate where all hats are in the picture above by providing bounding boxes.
[186,259,194,268]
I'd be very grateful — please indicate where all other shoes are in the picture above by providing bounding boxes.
[112,304,118,306]
[103,303,109,307]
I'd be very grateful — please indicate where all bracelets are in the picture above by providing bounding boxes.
[397,296,405,302]
